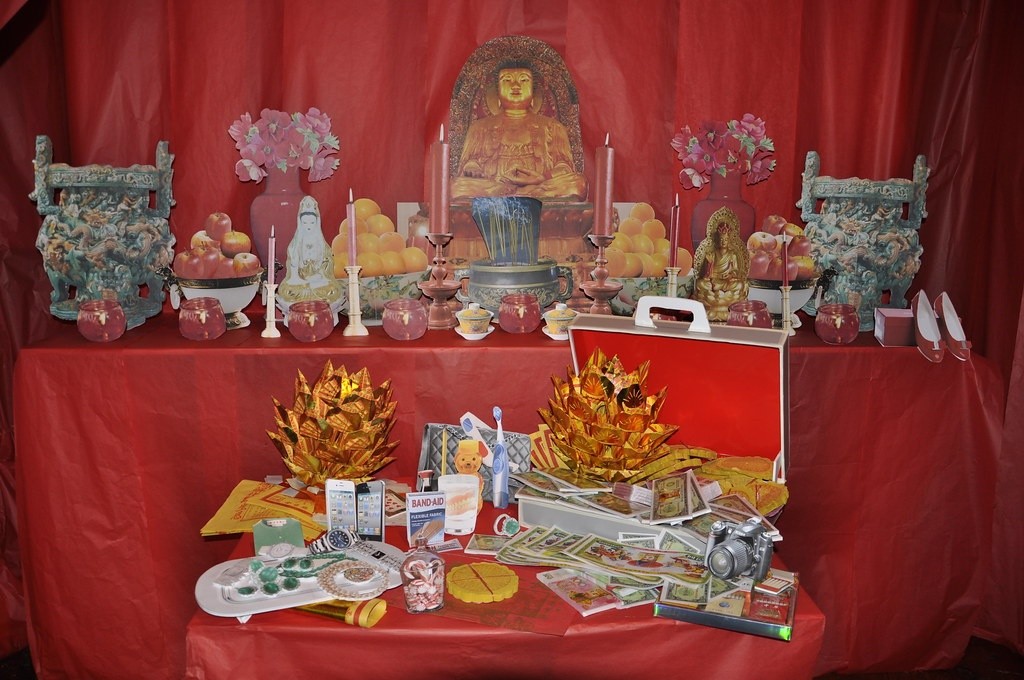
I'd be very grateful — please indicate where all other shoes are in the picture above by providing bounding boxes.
[912,289,947,363]
[931,292,972,362]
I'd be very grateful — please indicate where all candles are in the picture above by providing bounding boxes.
[428,124,450,233]
[268,225,276,284]
[783,231,788,286]
[669,193,680,267]
[593,133,615,235]
[346,188,357,266]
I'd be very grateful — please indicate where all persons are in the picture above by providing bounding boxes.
[451,59,587,201]
[285,196,333,288]
[704,233,738,281]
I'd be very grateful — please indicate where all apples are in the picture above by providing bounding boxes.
[173,209,260,280]
[746,216,815,281]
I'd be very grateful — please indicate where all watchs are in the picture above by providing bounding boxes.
[308,527,362,555]
[353,540,401,571]
[260,543,309,560]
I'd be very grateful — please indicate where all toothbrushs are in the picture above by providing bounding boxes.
[491,406,509,509]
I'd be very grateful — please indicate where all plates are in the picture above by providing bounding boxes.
[542,324,572,340]
[454,326,495,340]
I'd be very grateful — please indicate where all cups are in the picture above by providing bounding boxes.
[437,474,480,535]
[542,304,578,334]
[455,303,494,334]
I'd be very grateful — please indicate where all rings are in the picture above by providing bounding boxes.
[493,514,520,537]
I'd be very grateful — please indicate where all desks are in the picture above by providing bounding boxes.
[12,311,1024,680]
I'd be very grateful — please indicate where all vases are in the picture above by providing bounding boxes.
[691,173,755,254]
[251,166,309,283]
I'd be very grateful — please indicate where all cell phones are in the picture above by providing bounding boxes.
[325,479,385,543]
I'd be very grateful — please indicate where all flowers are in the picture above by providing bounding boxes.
[227,107,341,182]
[670,113,777,190]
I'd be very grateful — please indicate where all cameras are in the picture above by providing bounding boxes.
[703,516,773,582]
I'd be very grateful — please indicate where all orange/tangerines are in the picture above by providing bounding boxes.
[331,198,429,278]
[603,203,691,277]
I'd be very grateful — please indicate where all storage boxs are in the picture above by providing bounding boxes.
[873,308,962,347]
